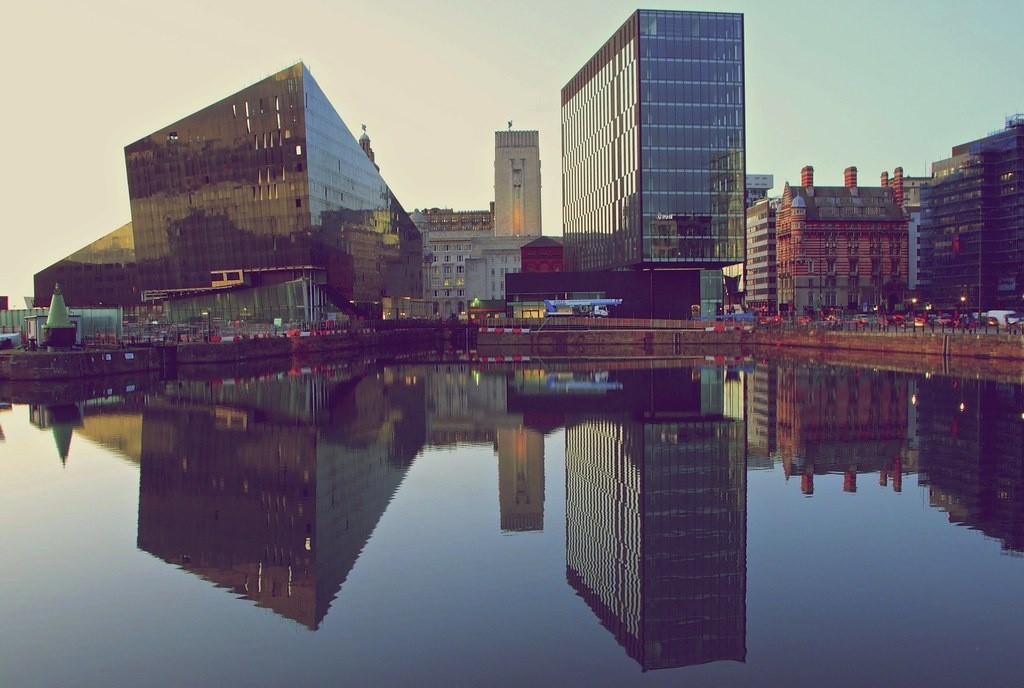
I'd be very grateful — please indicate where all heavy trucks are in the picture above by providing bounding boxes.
[543,304,610,318]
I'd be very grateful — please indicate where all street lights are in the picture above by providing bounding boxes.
[960,297,966,333]
[912,299,916,333]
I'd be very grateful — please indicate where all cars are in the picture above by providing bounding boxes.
[852,314,869,323]
